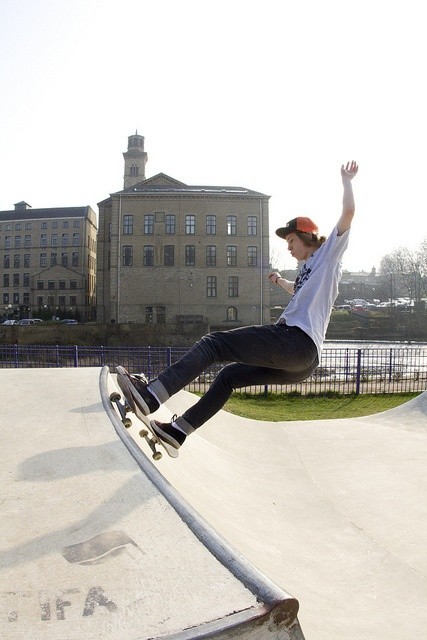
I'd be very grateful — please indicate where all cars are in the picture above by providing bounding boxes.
[4,320,17,326]
[18,319,35,325]
[352,305,366,310]
[344,298,369,305]
[62,319,77,325]
[338,305,350,310]
[373,297,415,307]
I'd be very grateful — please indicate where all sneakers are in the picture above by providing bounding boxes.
[151,414,186,448]
[116,366,159,416]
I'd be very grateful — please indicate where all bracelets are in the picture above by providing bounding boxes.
[274,277,283,285]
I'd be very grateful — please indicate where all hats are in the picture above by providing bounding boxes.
[276,217,319,239]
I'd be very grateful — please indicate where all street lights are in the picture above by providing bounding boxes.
[5,304,12,319]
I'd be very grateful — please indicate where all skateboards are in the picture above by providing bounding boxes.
[110,375,179,461]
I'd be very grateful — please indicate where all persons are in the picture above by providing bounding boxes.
[117,159,360,449]
